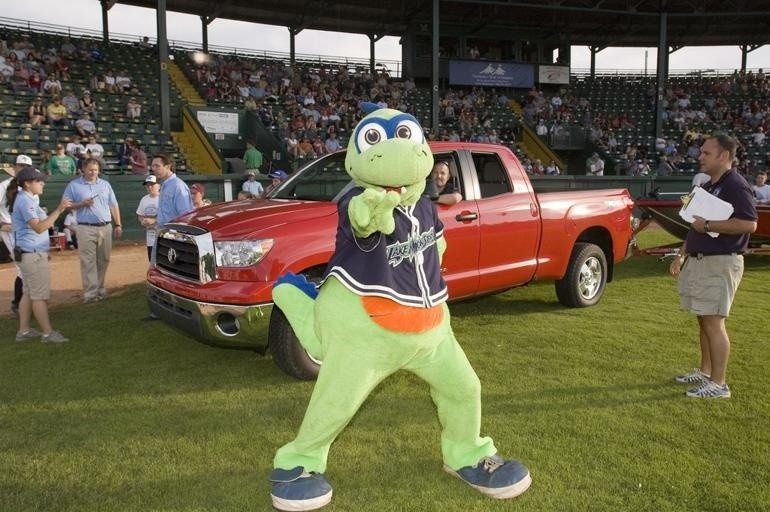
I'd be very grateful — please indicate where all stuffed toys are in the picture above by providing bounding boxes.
[267,102,534,512]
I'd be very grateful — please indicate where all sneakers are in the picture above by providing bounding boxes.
[686,381,730,399]
[675,368,711,384]
[42,331,70,343]
[16,330,41,339]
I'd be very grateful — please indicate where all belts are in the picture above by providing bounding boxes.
[690,251,744,257]
[78,222,109,226]
[22,251,31,254]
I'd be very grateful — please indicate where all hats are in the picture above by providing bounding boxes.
[18,168,49,181]
[83,90,91,94]
[14,155,32,166]
[248,172,255,177]
[142,175,159,185]
[129,97,137,100]
[191,183,204,193]
[71,135,82,139]
[89,135,96,140]
[57,144,64,149]
[268,170,288,180]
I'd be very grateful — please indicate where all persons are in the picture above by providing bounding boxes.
[5,167,73,343]
[1,22,769,266]
[139,154,193,322]
[0,155,40,314]
[670,134,759,400]
[63,157,123,304]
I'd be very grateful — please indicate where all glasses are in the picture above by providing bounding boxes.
[85,93,91,95]
[435,161,448,165]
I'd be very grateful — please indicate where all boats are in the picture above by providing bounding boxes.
[636,195,770,266]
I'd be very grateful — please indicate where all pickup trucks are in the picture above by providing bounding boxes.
[145,140,637,381]
